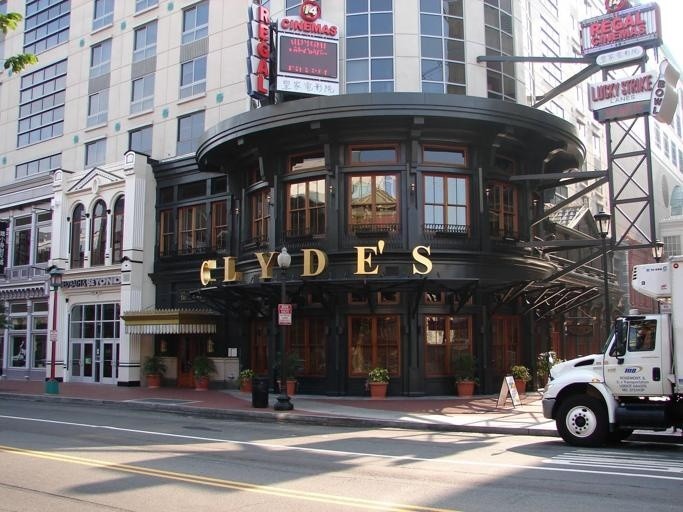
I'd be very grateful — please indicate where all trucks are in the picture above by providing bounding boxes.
[543,255,683,446]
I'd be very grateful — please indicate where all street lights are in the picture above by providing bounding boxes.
[651,239,664,314]
[274,246,295,409]
[44,264,66,394]
[594,207,613,336]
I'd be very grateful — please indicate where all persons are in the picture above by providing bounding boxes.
[633,323,653,350]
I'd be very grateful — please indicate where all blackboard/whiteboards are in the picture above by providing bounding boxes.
[505,376,522,406]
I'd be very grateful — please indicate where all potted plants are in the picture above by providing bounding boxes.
[449,351,483,395]
[139,350,169,389]
[509,349,559,395]
[238,350,305,396]
[366,363,391,398]
[192,354,218,391]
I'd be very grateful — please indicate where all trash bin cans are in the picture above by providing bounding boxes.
[252,376,270,408]
